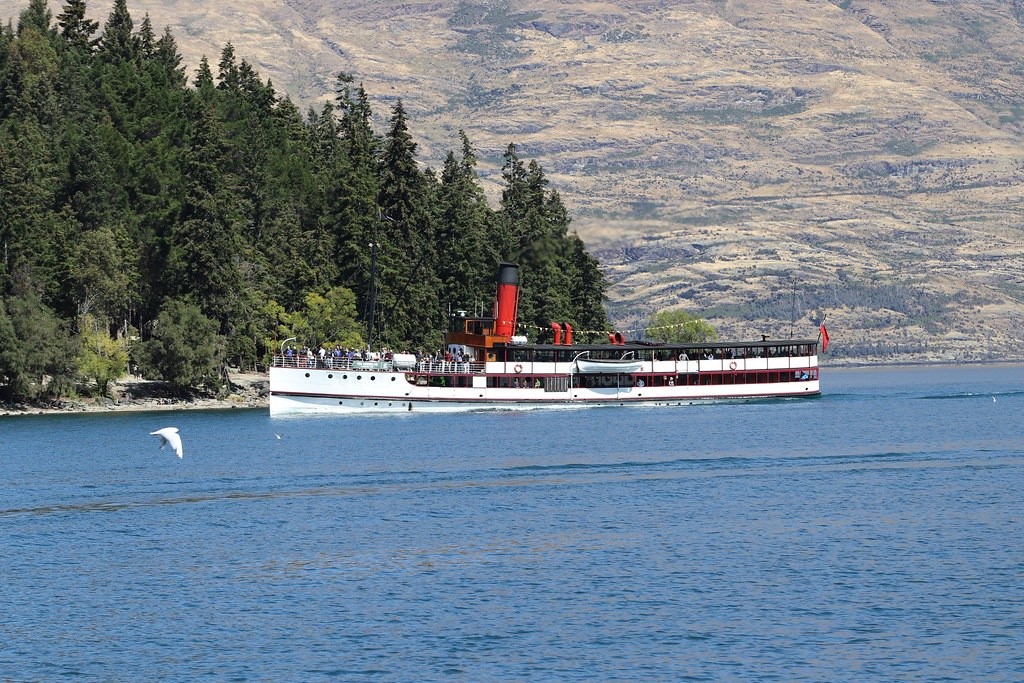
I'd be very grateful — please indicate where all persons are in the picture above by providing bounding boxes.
[286,345,293,367]
[668,377,674,386]
[708,353,714,360]
[325,348,332,368]
[679,350,689,361]
[712,349,733,359]
[522,380,529,388]
[292,347,298,367]
[299,346,326,368]
[534,378,540,388]
[359,345,393,372]
[334,345,361,368]
[399,350,435,372]
[649,349,708,361]
[637,379,645,387]
[748,348,796,358]
[435,350,475,374]
[514,378,520,388]
[801,372,808,380]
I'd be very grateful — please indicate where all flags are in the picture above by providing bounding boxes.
[819,323,829,353]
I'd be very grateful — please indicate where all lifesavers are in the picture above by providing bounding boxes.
[729,362,737,371]
[299,355,306,364]
[514,364,523,373]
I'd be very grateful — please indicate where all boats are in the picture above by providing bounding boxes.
[576,355,645,375]
[268,315,822,404]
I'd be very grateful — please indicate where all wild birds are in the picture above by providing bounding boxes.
[991,395,996,402]
[274,433,285,439]
[150,426,184,459]
